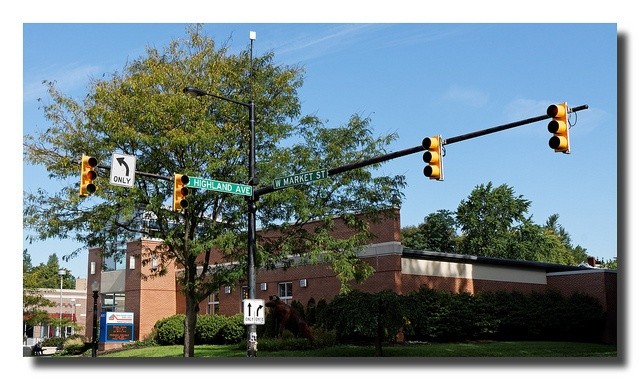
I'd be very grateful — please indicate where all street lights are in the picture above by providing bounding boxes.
[183,86,259,357]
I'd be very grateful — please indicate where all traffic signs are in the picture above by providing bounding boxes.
[243,299,265,326]
[109,153,136,188]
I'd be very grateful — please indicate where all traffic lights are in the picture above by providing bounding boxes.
[547,102,568,154]
[173,172,189,212]
[422,135,441,180]
[79,154,97,197]
